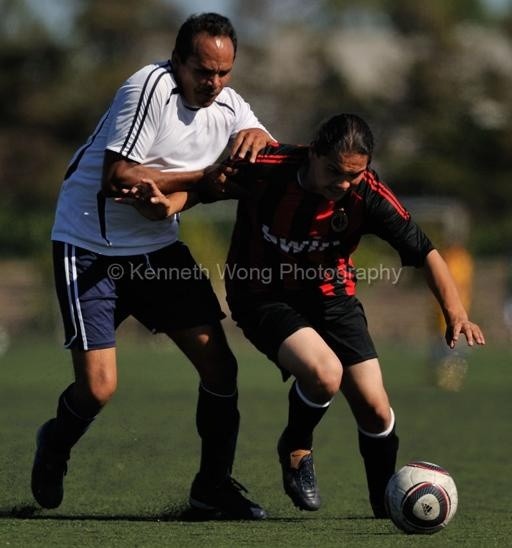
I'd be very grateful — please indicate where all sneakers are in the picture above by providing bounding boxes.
[275,432,322,512]
[185,472,264,523]
[355,437,402,516]
[30,417,69,511]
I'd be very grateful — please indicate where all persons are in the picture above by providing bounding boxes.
[111,112,487,519]
[29,10,282,521]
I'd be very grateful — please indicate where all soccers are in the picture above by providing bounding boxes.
[387,461,457,533]
